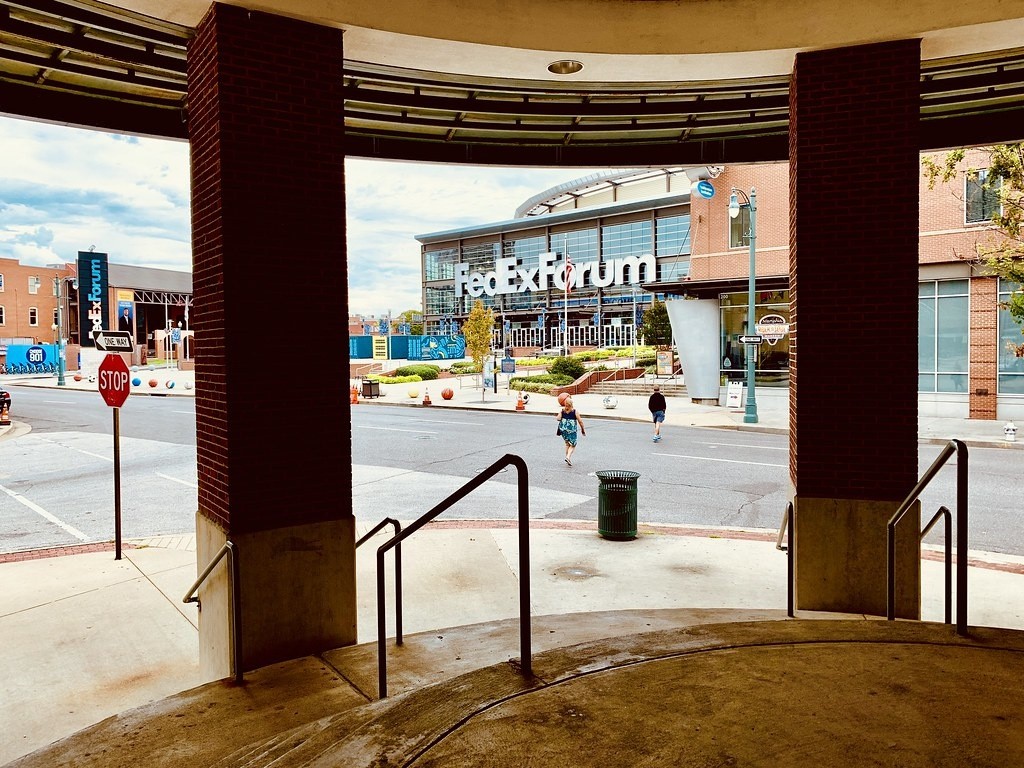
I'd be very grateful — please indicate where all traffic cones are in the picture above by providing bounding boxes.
[422,387,432,405]
[0,403,11,426]
[515,391,525,410]
[349,384,360,404]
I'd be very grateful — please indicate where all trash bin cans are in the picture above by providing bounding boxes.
[362,379,379,399]
[596,470,642,540]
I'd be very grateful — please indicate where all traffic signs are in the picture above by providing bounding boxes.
[736,334,763,345]
[92,331,134,353]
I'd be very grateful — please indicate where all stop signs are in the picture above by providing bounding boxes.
[97,352,131,408]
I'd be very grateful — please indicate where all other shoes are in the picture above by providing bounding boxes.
[565,458,572,466]
[658,435,661,440]
[653,436,658,441]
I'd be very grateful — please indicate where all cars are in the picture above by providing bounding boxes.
[0,386,12,414]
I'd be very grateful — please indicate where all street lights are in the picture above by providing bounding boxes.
[166,319,176,368]
[34,273,80,387]
[726,185,761,424]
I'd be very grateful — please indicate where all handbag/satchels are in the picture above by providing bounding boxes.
[556,427,561,436]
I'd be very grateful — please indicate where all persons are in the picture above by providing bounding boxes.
[556,399,586,467]
[648,385,666,443]
